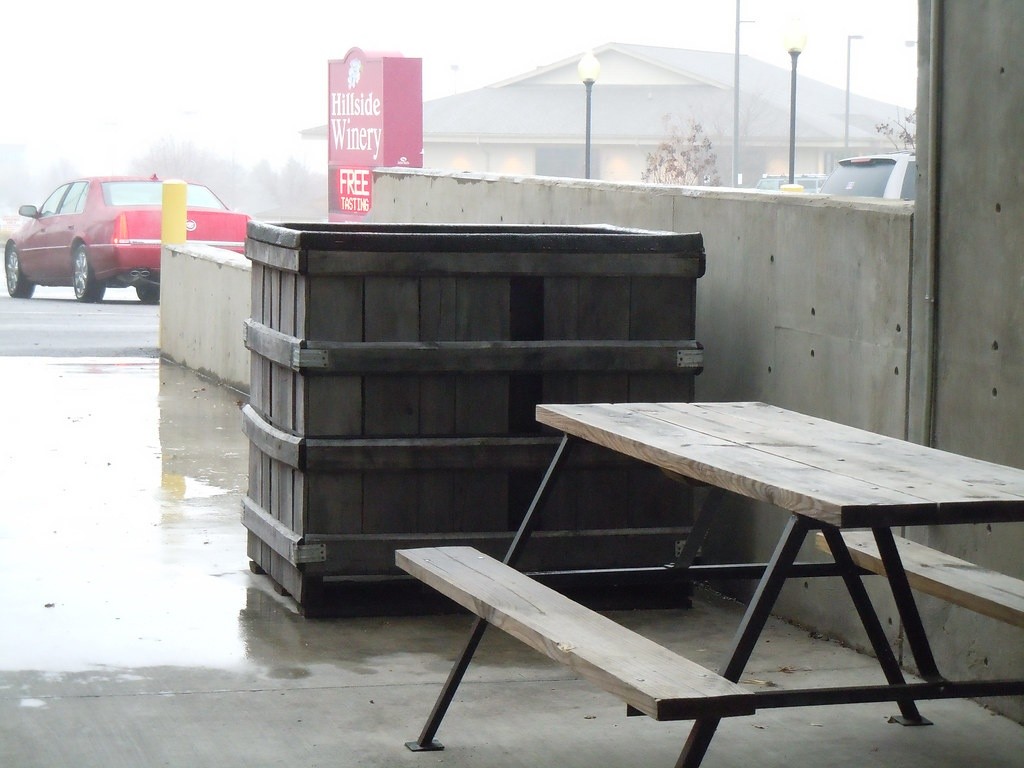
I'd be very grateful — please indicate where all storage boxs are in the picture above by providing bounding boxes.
[243,219,706,607]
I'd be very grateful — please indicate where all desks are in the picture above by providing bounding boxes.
[404,400,1024,768]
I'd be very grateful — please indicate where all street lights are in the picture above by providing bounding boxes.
[580,72,597,182]
[787,46,802,184]
[843,34,863,160]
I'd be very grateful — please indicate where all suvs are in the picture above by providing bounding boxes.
[754,173,828,190]
[818,150,916,201]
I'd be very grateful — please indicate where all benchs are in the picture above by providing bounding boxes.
[815,531,1024,631]
[394,544,758,722]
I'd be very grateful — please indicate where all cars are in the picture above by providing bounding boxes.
[4,176,252,302]
